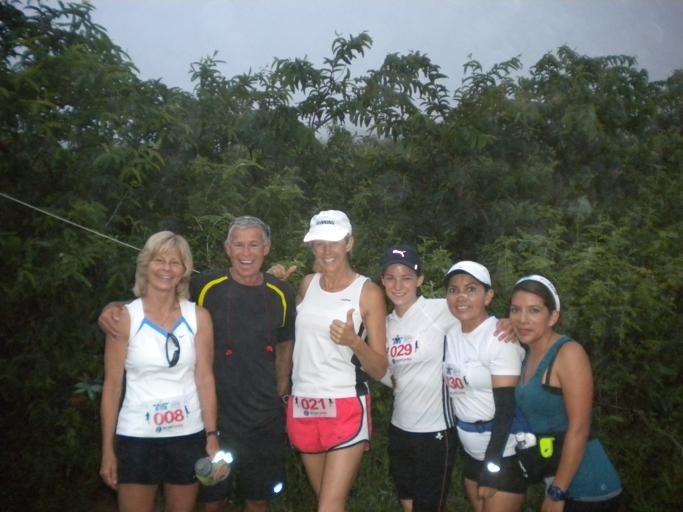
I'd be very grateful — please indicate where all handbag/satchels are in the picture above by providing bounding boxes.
[512,431,576,486]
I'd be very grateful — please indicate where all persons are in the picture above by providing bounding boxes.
[509,275,624,512]
[442,259,528,511]
[98,216,297,511]
[364,243,521,511]
[97,231,223,509]
[263,210,389,512]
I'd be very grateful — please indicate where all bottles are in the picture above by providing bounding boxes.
[193,457,229,487]
[515,431,531,450]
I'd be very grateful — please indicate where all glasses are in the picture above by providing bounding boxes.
[164,331,182,370]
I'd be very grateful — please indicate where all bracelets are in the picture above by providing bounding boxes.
[205,430,220,436]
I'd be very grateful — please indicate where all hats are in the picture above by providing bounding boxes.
[303,209,352,243]
[382,244,419,278]
[444,260,491,289]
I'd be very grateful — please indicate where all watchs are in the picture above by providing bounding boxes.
[545,485,569,502]
[279,394,290,404]
